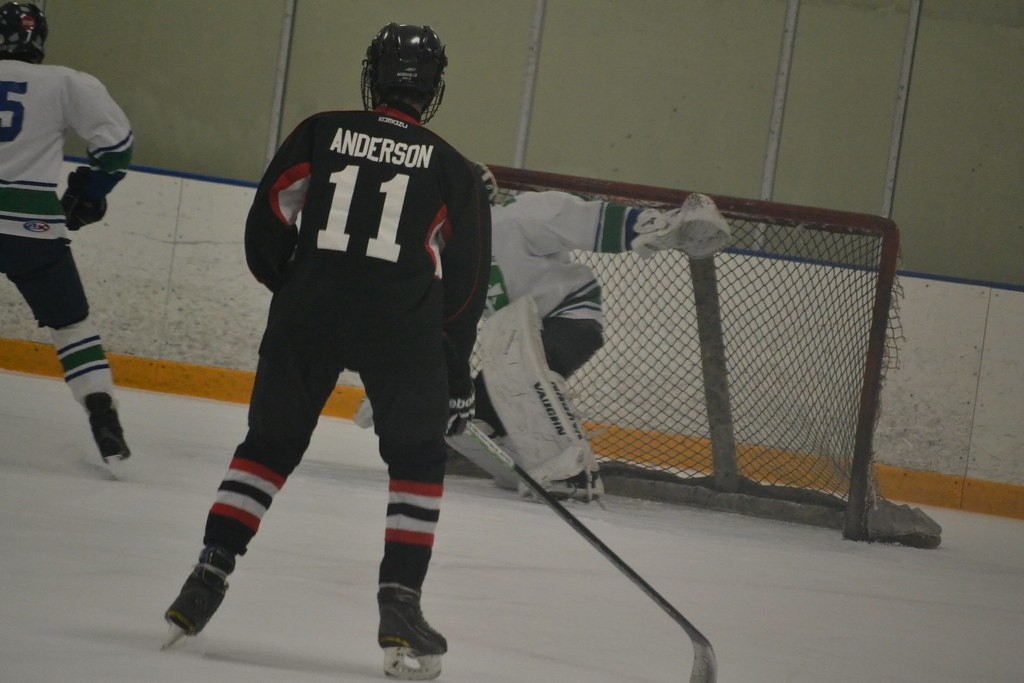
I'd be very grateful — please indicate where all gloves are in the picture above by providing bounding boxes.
[61,166,126,231]
[446,364,476,436]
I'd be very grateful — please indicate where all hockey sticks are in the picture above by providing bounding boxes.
[463,418,720,682]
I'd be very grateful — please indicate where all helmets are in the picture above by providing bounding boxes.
[0,1,47,61]
[360,22,448,125]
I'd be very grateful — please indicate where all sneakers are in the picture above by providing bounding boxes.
[550,469,604,498]
[159,544,235,649]
[378,588,448,681]
[85,391,131,480]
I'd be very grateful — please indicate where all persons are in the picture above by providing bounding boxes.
[354,163,731,507]
[0,0,138,479]
[159,23,491,681]
